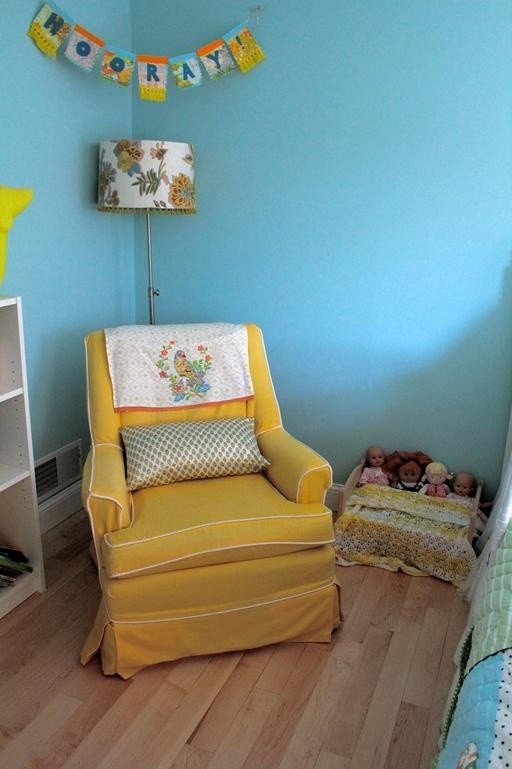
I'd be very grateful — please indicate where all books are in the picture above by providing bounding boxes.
[1,545,34,587]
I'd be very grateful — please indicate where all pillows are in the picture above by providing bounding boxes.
[117,415,271,492]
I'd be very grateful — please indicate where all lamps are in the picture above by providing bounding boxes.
[97,139,196,323]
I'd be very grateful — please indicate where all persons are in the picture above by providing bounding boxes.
[445,469,476,512]
[418,461,453,497]
[358,443,390,485]
[383,447,432,493]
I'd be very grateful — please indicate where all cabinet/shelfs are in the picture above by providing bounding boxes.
[1,294,48,617]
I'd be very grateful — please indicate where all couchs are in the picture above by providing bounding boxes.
[80,323,344,680]
